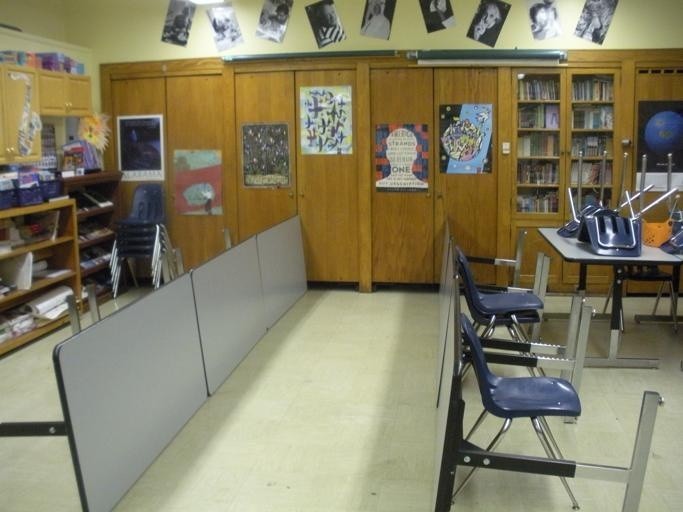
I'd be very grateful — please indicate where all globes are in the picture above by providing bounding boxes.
[644,111,683,166]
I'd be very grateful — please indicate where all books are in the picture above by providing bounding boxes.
[518,74,614,214]
[0,141,114,343]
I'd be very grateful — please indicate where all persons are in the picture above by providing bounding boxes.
[469,2,504,47]
[173,5,192,31]
[529,0,558,42]
[263,3,290,35]
[312,0,346,49]
[359,0,390,41]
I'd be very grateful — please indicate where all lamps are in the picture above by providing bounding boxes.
[414,49,567,69]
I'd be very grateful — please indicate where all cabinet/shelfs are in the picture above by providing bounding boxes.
[0,171,122,357]
[0,63,91,165]
[508,67,621,293]
[98,51,496,292]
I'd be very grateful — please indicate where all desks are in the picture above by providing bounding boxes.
[537,228,683,369]
[432,221,664,512]
[0,213,307,512]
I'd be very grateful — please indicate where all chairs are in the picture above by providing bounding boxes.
[454,247,581,512]
[108,183,175,298]
[556,149,683,257]
